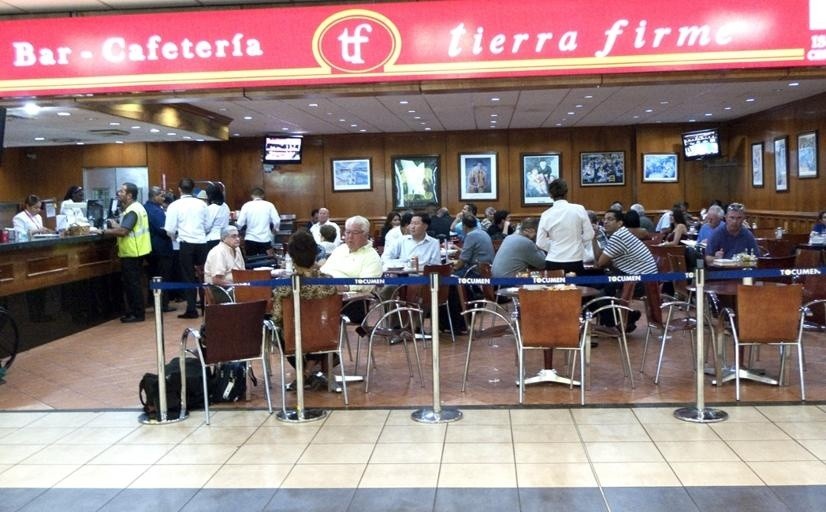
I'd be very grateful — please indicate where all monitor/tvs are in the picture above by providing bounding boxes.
[682,128,720,161]
[110,199,119,213]
[263,134,303,164]
[87,198,104,220]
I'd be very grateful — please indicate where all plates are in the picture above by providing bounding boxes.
[34,233,59,237]
[252,266,274,271]
[386,265,404,270]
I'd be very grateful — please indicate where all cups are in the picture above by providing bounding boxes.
[690,226,695,233]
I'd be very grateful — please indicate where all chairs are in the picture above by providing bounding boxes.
[173,212,825,429]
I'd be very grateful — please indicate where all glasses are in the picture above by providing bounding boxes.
[728,205,743,210]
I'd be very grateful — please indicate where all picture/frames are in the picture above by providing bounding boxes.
[751,141,764,189]
[796,128,819,180]
[639,152,679,183]
[330,157,374,194]
[577,149,627,187]
[390,153,442,212]
[457,150,499,203]
[519,150,563,209]
[773,134,790,193]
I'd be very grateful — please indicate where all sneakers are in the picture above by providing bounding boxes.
[290,374,327,390]
[627,311,640,332]
[595,325,620,337]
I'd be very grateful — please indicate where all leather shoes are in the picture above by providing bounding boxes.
[179,310,198,318]
[162,305,178,311]
[120,313,145,322]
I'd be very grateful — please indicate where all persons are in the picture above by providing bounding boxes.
[160,190,178,212]
[143,183,176,314]
[203,180,546,390]
[806,210,826,248]
[582,199,758,333]
[90,183,156,323]
[10,194,54,238]
[165,178,207,321]
[536,179,599,348]
[57,183,88,219]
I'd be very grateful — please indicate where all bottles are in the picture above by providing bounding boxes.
[411,256,419,272]
[284,253,294,273]
[749,248,756,265]
[776,226,785,239]
[695,246,703,268]
[752,222,758,238]
[741,247,749,266]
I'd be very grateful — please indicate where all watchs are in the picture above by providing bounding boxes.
[102,230,105,235]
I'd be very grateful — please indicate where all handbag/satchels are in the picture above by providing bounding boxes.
[140,358,246,414]
